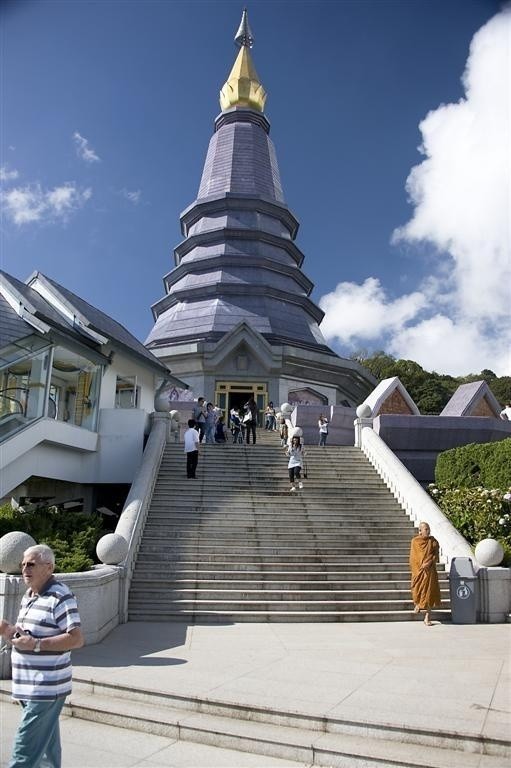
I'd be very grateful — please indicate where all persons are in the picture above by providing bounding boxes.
[284,436,305,492]
[183,419,201,479]
[191,397,288,447]
[408,522,441,626]
[318,413,329,446]
[0,545,84,768]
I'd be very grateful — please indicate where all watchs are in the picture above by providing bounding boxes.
[34,639,41,653]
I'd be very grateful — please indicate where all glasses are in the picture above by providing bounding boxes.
[19,562,50,568]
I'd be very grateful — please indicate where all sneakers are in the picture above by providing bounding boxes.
[289,486,296,492]
[299,482,303,489]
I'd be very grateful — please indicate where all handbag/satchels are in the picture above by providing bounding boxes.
[304,474,307,478]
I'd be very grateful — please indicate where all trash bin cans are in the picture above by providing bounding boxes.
[446,557,479,624]
